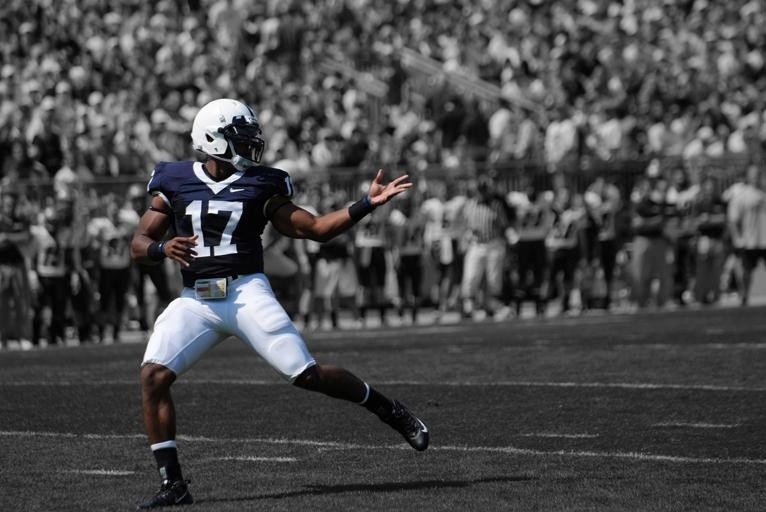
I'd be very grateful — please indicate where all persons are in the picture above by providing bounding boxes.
[0,0,765,352]
[122,96,432,512]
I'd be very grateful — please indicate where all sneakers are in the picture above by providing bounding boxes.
[380,398,430,452]
[137,479,194,509]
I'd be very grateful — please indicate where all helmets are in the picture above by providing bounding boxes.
[191,97,259,155]
